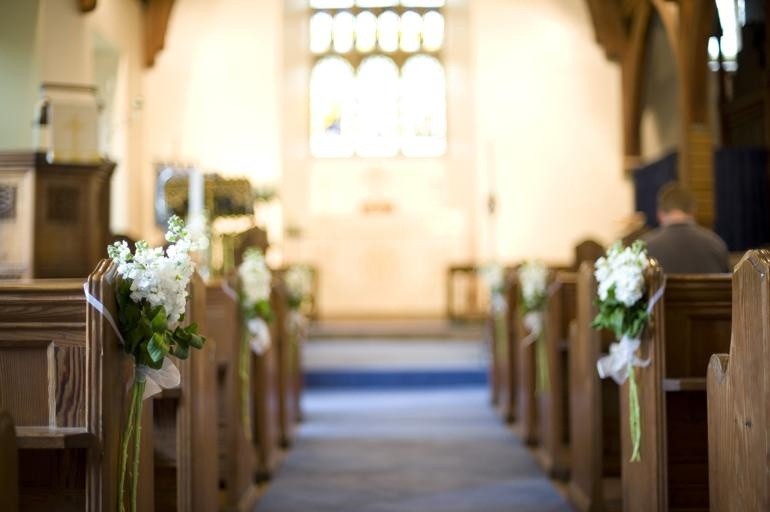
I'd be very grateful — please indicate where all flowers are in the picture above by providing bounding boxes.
[102,212,205,372]
[594,239,658,339]
[238,247,319,355]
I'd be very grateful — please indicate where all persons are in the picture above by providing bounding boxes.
[628,181,731,274]
